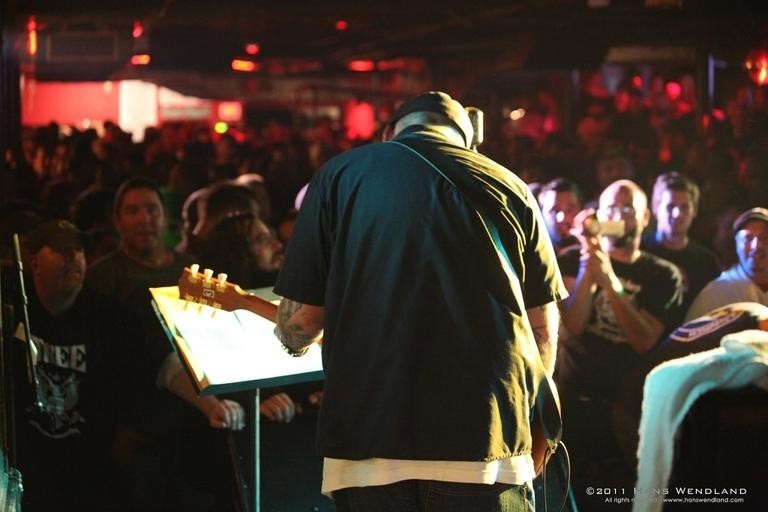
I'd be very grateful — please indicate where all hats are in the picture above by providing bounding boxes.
[387,89,473,148]
[731,207,767,233]
[28,219,90,255]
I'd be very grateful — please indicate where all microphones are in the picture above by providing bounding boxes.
[25,372,49,415]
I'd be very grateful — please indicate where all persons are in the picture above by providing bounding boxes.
[1,73,768,511]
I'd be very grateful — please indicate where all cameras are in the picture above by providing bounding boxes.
[584,217,626,238]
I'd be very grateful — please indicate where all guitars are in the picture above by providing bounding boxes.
[178,263,556,481]
